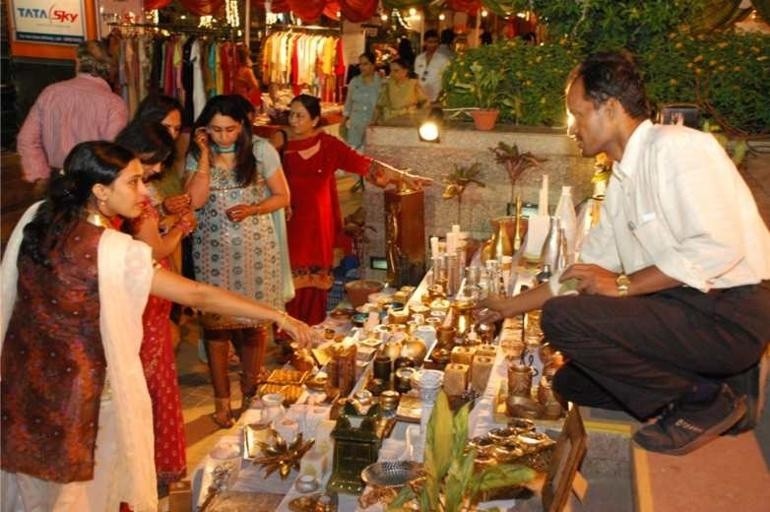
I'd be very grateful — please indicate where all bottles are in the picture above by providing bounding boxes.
[463,256,514,340]
[532,186,580,286]
[243,387,407,459]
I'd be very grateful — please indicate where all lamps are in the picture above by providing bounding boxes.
[421,107,445,144]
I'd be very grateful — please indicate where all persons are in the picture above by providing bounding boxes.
[108,119,197,498]
[136,94,188,269]
[372,59,431,126]
[185,93,291,427]
[342,53,383,180]
[0,140,327,512]
[522,32,539,47]
[17,42,128,199]
[459,47,770,455]
[478,31,492,45]
[274,95,434,364]
[396,29,454,100]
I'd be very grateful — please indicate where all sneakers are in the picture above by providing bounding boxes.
[633,384,746,455]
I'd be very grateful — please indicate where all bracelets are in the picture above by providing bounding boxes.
[278,312,288,332]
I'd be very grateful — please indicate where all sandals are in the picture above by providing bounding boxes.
[213,399,234,427]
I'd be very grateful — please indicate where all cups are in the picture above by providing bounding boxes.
[465,398,559,466]
[505,365,533,398]
[373,356,390,379]
[288,474,340,511]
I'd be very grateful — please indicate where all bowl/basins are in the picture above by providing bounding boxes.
[345,280,384,307]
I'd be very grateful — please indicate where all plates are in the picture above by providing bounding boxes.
[362,460,419,490]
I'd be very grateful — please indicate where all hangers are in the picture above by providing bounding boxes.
[266,23,343,47]
[104,17,239,55]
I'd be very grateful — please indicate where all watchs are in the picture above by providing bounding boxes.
[614,269,632,298]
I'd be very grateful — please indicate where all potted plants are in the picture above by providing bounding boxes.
[486,143,547,253]
[439,160,488,269]
[462,61,513,131]
